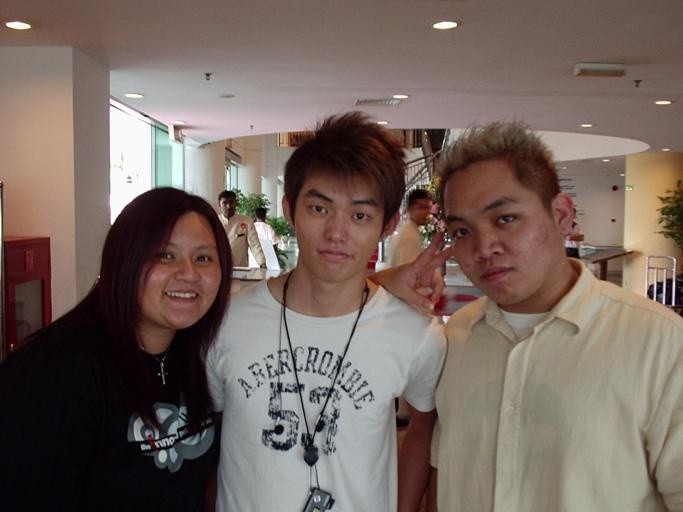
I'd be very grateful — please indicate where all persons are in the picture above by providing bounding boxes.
[215,190,267,268]
[1,186,454,512]
[426,114,683,512]
[248,207,281,270]
[561,207,584,259]
[388,188,434,266]
[199,109,449,511]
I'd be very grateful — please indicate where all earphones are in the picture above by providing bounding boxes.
[275,413,283,434]
[316,414,324,432]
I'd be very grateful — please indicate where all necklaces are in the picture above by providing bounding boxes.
[139,341,171,385]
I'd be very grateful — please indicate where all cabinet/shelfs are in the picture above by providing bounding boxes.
[1,232,53,353]
